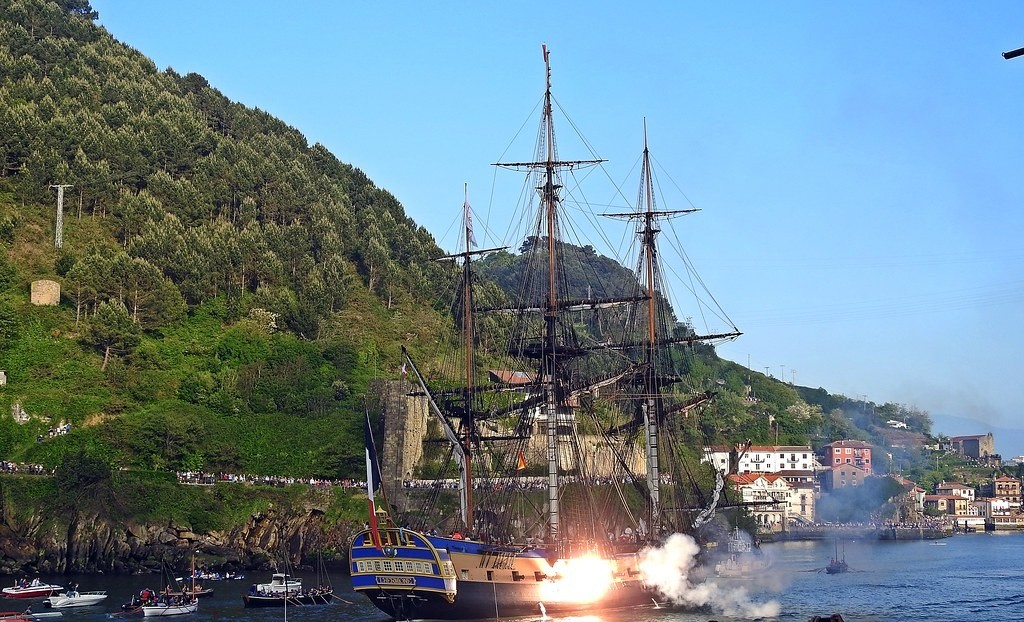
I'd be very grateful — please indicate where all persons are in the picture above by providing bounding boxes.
[19,577,40,588]
[528,534,544,549]
[403,479,458,490]
[660,470,670,484]
[66,581,81,599]
[37,422,72,442]
[452,529,463,540]
[257,584,331,598]
[365,521,369,528]
[21,604,33,615]
[607,521,643,544]
[560,472,648,484]
[405,523,437,537]
[0,458,58,476]
[194,562,236,580]
[131,584,203,606]
[170,468,368,490]
[472,478,549,492]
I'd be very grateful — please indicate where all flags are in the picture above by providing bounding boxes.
[514,449,527,470]
[465,201,478,248]
[363,403,386,556]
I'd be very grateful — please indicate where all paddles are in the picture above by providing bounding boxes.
[184,605,192,615]
[320,595,330,605]
[286,599,299,606]
[294,598,304,605]
[329,593,353,605]
[178,607,185,615]
[311,596,317,607]
[159,609,167,616]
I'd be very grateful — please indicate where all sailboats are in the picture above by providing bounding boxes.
[349,39,788,621]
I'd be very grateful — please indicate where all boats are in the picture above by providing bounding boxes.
[982,514,1024,532]
[824,537,847,574]
[108,552,245,616]
[242,524,334,607]
[0,582,108,622]
